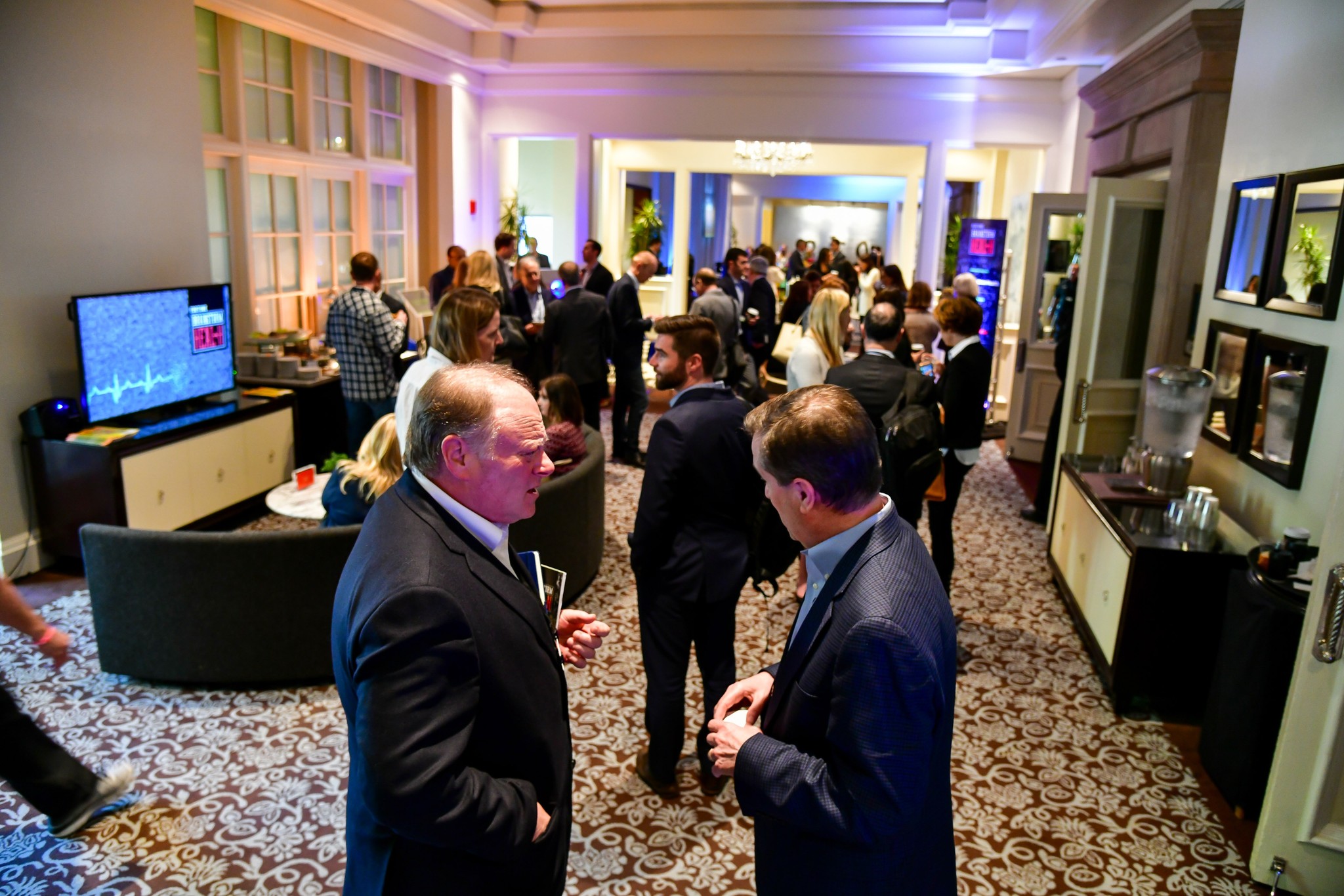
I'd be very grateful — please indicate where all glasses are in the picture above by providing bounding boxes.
[691,282,699,292]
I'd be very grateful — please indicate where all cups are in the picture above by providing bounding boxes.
[747,307,759,321]
[1279,526,1311,573]
[1162,486,1218,533]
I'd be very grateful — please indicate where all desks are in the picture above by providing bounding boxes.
[1196,570,1306,826]
[234,349,419,471]
[265,472,330,519]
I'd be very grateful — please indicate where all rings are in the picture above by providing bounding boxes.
[707,748,715,761]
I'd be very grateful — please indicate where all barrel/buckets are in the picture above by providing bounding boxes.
[1128,365,1215,494]
[1263,369,1305,465]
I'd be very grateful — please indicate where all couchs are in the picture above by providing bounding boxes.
[79,423,606,684]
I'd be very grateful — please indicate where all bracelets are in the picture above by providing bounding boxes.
[33,626,53,645]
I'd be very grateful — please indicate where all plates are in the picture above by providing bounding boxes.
[235,352,321,381]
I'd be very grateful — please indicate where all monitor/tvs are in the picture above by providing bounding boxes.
[71,283,238,427]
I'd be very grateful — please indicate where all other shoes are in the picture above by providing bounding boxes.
[699,761,729,797]
[620,441,647,468]
[612,448,648,465]
[1019,508,1046,524]
[636,746,679,800]
[45,764,133,839]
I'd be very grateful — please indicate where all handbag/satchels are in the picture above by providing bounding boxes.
[771,322,803,364]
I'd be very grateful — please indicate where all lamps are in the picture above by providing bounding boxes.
[18,396,83,440]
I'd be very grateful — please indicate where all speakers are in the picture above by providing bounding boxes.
[17,397,80,441]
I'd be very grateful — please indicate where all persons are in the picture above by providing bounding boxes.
[707,385,958,896]
[0,569,132,838]
[394,288,503,470]
[537,374,589,479]
[647,237,663,274]
[331,362,612,896]
[1213,276,1326,403]
[689,268,741,385]
[928,297,991,598]
[325,252,410,447]
[323,413,404,526]
[823,302,937,528]
[430,232,613,428]
[607,251,658,468]
[1020,264,1079,529]
[716,239,978,386]
[785,289,851,390]
[636,316,770,804]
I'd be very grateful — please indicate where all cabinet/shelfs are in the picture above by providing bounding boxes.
[28,389,302,560]
[1046,453,1263,727]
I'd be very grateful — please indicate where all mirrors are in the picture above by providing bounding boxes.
[1212,173,1285,308]
[1238,332,1330,491]
[1200,319,1262,454]
[1262,163,1344,322]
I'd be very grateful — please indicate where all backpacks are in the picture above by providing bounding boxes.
[743,471,804,596]
[876,370,942,505]
